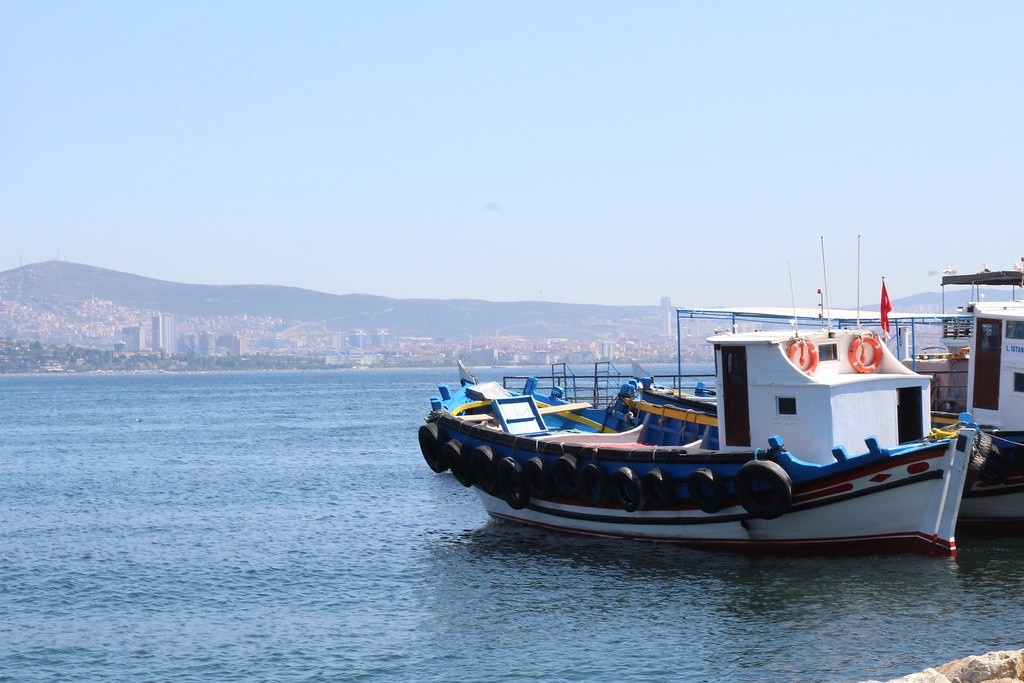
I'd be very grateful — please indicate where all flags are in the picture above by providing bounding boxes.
[881,283,892,332]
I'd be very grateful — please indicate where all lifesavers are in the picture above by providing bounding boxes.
[418,423,448,474]
[576,463,608,508]
[549,454,578,499]
[609,467,642,512]
[500,481,531,511]
[469,445,498,484]
[495,457,524,484]
[785,338,820,377]
[641,468,676,512]
[685,467,727,514]
[734,460,792,520]
[442,437,473,487]
[525,456,550,492]
[848,336,883,374]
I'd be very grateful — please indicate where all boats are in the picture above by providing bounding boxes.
[417,236,977,560]
[500,255,1023,535]
